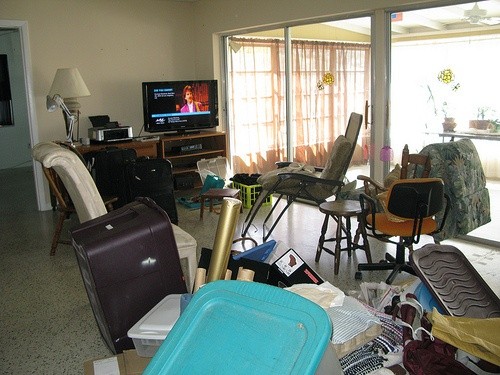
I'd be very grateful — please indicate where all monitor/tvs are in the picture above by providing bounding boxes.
[141,79,219,136]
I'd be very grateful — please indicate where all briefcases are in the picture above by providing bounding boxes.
[83,146,138,195]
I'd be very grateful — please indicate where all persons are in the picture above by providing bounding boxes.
[176,85,204,113]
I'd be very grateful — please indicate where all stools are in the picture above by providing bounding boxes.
[315,199,373,276]
[199,189,243,221]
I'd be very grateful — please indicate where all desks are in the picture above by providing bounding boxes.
[439,128,500,142]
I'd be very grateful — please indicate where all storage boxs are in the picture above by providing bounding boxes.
[232,180,273,208]
[68,196,345,375]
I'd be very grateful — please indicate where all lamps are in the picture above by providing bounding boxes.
[464,1,487,24]
[437,69,460,91]
[317,72,334,90]
[46,94,77,148]
[228,35,240,53]
[49,67,91,146]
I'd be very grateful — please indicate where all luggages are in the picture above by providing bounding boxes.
[121,156,178,228]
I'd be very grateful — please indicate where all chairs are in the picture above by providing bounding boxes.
[355,177,450,286]
[32,140,196,293]
[241,112,362,241]
[42,143,118,257]
[348,137,490,254]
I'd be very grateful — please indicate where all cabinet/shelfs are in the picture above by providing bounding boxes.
[53,135,159,191]
[155,131,229,192]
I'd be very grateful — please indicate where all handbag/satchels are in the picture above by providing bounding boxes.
[198,175,225,203]
[391,292,499,375]
[428,305,500,365]
